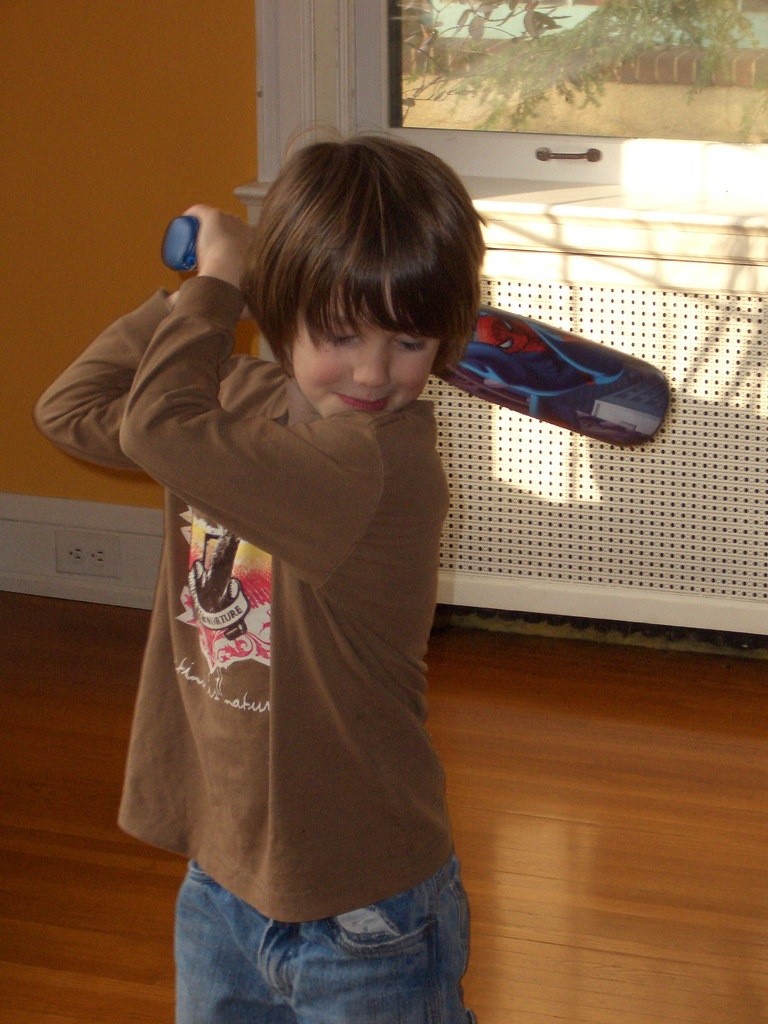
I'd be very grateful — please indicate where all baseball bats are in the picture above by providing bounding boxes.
[159,216,673,450]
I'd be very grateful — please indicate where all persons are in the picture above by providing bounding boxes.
[31,136,490,1024]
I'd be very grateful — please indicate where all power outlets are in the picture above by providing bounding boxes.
[53,528,118,577]
